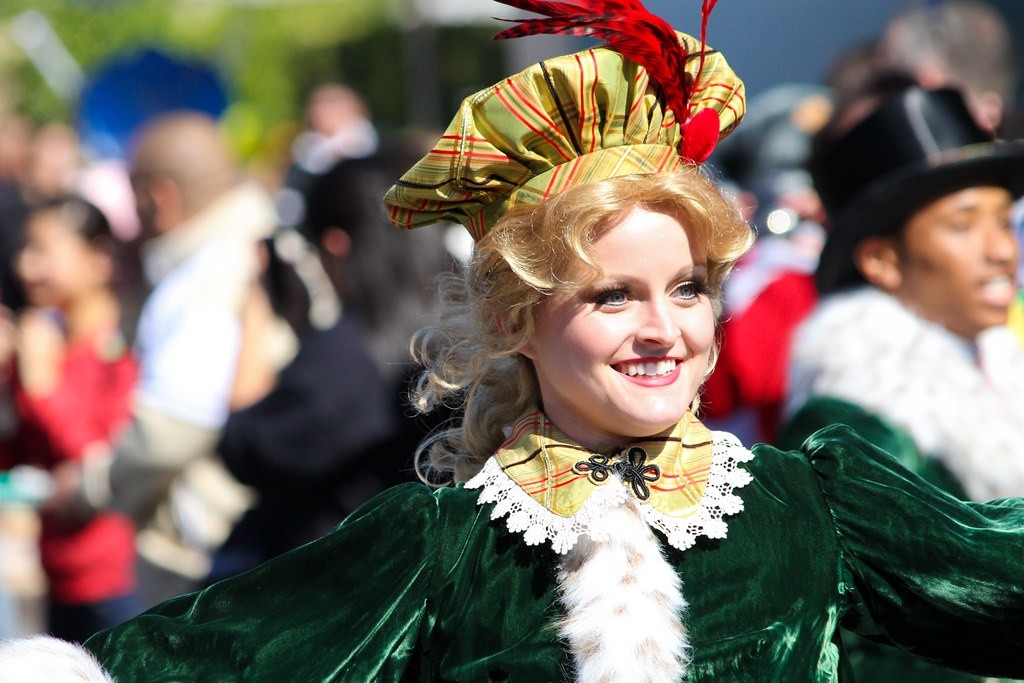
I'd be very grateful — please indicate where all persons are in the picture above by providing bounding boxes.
[872,4,1022,289]
[17,116,143,242]
[282,78,410,247]
[2,30,1024,681]
[0,197,268,650]
[3,112,400,570]
[700,111,828,451]
[783,85,1023,503]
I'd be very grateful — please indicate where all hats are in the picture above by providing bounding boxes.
[383,1,746,244]
[803,87,1024,297]
